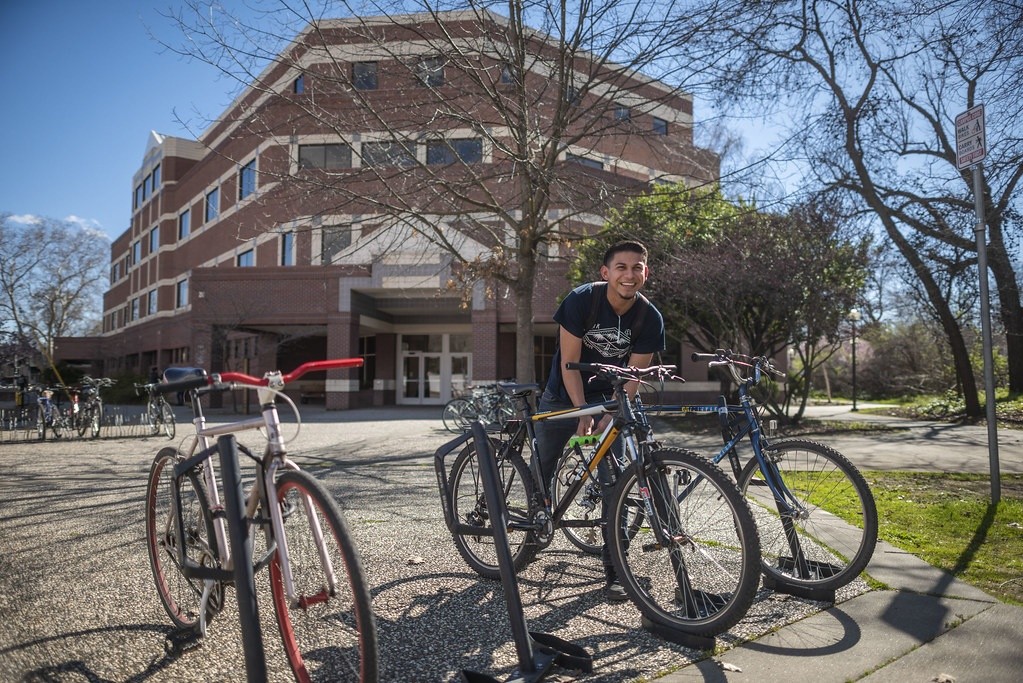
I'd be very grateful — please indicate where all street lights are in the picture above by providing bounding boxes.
[848,309,861,411]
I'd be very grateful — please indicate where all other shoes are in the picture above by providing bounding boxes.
[532,491,552,513]
[603,576,652,601]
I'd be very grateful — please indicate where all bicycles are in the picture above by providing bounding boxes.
[550,349,878,597]
[143,358,379,683]
[133,380,176,439]
[441,380,515,435]
[20,374,117,440]
[446,362,763,639]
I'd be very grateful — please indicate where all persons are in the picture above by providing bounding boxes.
[527,240,666,599]
